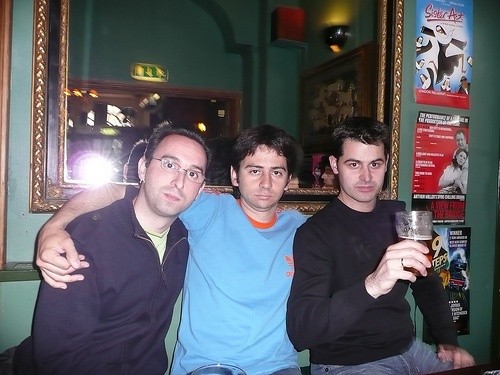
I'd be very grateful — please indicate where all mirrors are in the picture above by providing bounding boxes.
[32,0,403,213]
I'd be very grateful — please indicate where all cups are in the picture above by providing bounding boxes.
[393,212,435,276]
[191,364,246,375]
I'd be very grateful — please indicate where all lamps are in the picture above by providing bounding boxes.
[323,24,350,51]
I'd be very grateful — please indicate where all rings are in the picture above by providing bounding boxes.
[400,257,405,267]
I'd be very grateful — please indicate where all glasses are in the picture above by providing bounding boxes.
[152,157,208,184]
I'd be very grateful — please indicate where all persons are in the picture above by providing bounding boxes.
[32,126,212,375]
[321,157,338,190]
[124,139,147,184]
[438,129,468,194]
[35,121,308,374]
[457,76,470,95]
[286,115,478,375]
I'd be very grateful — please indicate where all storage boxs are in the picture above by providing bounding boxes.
[271,5,306,42]
